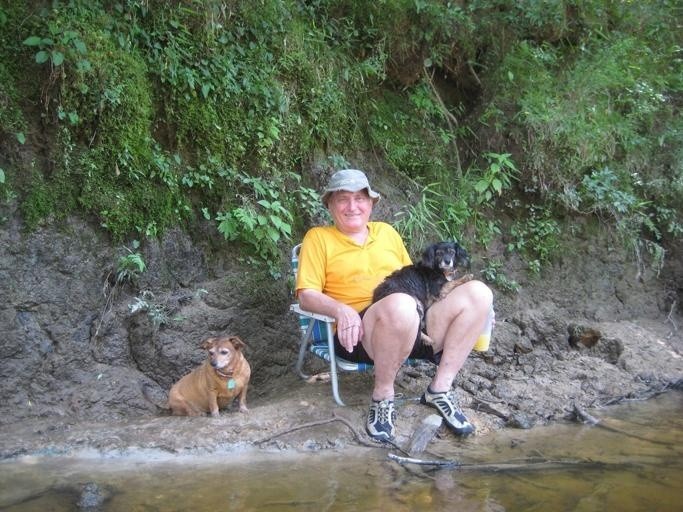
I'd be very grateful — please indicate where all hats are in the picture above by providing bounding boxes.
[322,168,379,209]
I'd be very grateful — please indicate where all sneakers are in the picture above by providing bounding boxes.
[419,386,475,434]
[367,396,396,442]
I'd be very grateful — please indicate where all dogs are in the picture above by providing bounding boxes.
[141,335,253,421]
[371,241,473,360]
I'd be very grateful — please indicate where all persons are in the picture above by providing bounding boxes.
[291,168,494,443]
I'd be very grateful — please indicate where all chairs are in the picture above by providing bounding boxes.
[288,240,438,409]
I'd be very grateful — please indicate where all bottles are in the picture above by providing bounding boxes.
[474,302,493,352]
